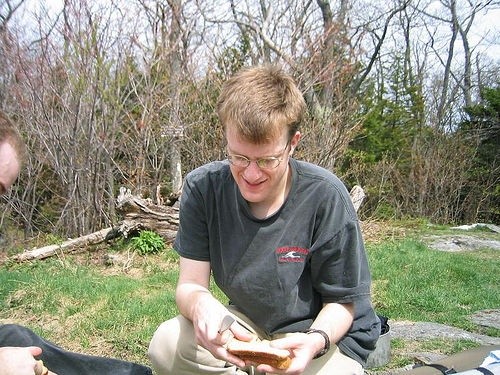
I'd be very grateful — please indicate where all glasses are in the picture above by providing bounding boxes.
[224,137,291,170]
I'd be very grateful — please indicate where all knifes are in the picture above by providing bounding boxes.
[214,314,236,346]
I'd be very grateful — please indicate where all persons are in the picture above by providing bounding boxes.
[0,113,152,375]
[147,64,381,375]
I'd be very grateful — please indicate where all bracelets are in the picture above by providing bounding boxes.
[305,329,330,360]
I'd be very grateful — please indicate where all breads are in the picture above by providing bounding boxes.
[226,337,292,370]
